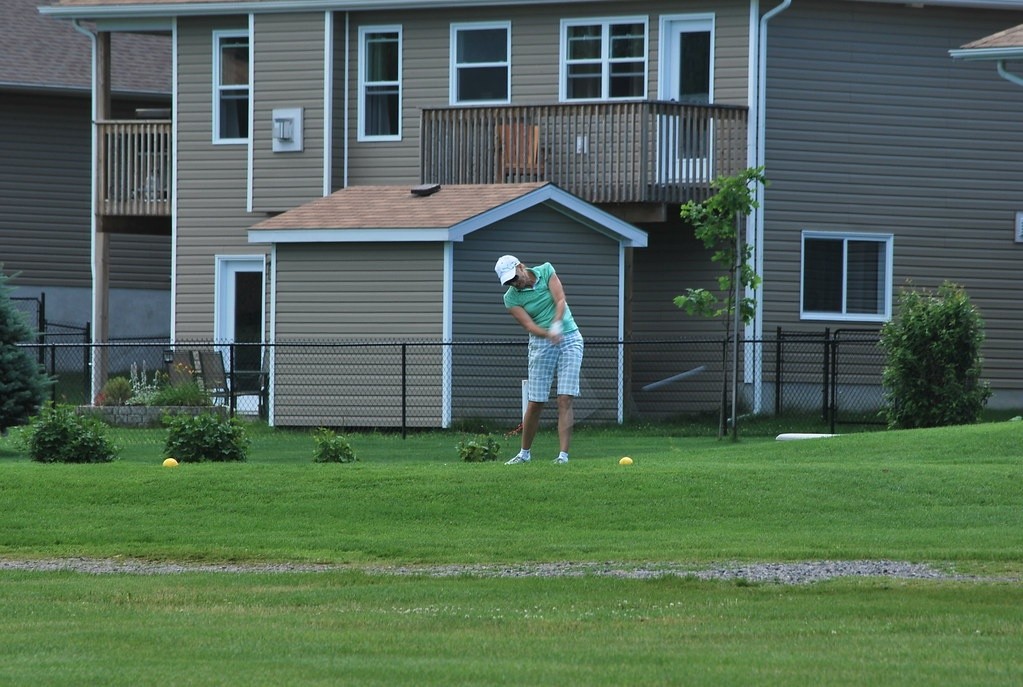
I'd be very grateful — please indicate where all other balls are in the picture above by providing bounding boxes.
[619,457,633,465]
[163,458,178,467]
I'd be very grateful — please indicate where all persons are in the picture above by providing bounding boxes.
[494,255,584,465]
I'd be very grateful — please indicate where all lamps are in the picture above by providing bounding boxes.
[274,118,294,144]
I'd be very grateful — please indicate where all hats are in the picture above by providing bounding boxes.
[495,255,521,287]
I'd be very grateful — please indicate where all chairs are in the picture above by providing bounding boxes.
[493,117,552,182]
[163,348,269,417]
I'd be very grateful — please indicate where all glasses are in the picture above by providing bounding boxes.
[505,266,520,286]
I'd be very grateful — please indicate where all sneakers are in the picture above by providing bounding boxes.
[553,455,568,464]
[504,454,531,465]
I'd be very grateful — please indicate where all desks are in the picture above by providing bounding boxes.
[195,370,268,416]
